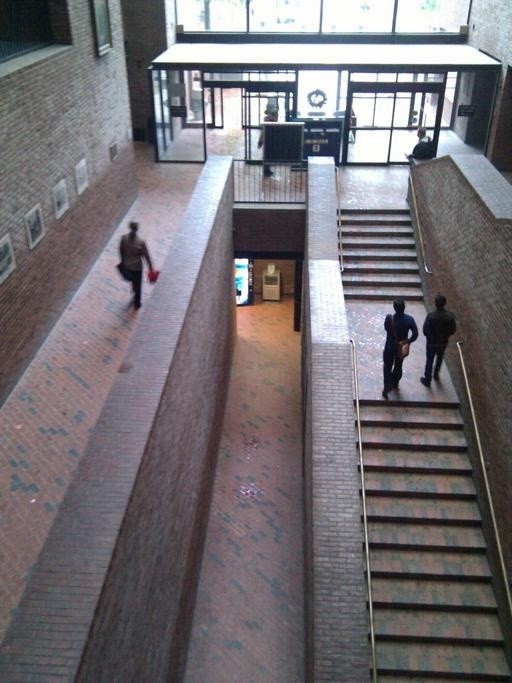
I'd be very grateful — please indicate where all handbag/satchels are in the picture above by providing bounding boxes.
[398,339,410,357]
[117,262,127,281]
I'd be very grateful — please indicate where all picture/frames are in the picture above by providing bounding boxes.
[91,0,114,57]
[1,158,91,284]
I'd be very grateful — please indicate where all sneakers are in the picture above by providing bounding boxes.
[420,378,430,389]
[380,387,392,397]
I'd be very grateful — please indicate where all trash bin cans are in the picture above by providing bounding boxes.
[191,88,205,121]
[262,270,280,301]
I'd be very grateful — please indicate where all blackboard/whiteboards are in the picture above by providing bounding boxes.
[263,122,304,166]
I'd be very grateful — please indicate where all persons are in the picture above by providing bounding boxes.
[118,221,155,312]
[256,99,279,179]
[381,299,420,397]
[420,296,456,386]
[405,127,438,163]
